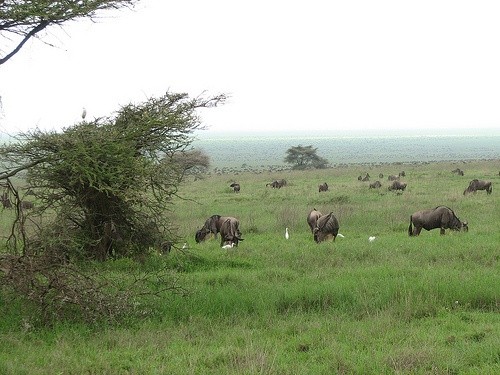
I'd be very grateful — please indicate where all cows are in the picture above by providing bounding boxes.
[407,206,469,236]
[307,208,339,243]
[463,179,492,195]
[358,171,407,192]
[452,169,463,176]
[318,183,328,192]
[196,215,244,249]
[270,179,287,189]
[230,183,240,192]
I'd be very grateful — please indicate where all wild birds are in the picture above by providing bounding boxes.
[222,243,236,250]
[285,228,289,239]
[183,243,188,249]
[368,236,375,243]
[332,234,344,238]
